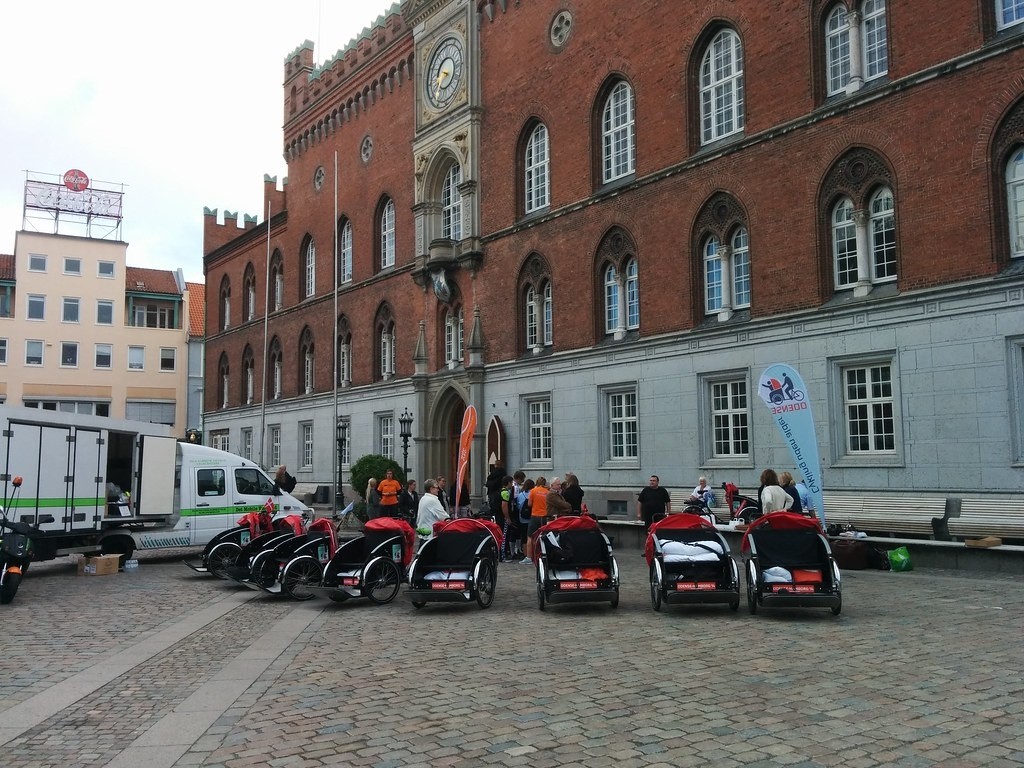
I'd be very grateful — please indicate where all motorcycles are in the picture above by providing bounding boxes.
[0,509,55,604]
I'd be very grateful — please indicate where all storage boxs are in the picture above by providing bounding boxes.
[78,554,124,576]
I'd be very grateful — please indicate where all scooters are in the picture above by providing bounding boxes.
[681,482,759,524]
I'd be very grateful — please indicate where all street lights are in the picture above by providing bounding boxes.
[398,407,414,482]
[333,419,347,521]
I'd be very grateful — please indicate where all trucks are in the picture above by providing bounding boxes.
[0,404,315,568]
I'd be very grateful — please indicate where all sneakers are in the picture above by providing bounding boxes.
[519,558,533,564]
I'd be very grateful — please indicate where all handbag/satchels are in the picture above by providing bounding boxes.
[706,497,716,508]
[860,544,891,571]
[729,518,745,526]
[887,546,913,572]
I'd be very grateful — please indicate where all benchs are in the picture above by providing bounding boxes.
[647,528,731,563]
[948,499,1024,540]
[250,528,293,555]
[218,523,264,540]
[275,531,331,563]
[334,530,403,566]
[598,521,1024,573]
[417,531,492,569]
[539,529,613,567]
[747,530,832,567]
[291,483,319,505]
[664,490,946,540]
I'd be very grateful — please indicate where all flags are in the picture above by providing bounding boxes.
[265,498,275,512]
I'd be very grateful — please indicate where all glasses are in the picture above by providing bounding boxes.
[433,486,439,489]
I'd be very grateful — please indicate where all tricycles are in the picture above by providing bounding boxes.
[250,518,354,601]
[182,510,309,579]
[741,511,842,615]
[531,514,619,611]
[402,515,504,609]
[644,513,740,611]
[305,517,418,604]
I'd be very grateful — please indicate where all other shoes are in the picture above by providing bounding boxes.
[503,558,513,562]
[641,554,646,557]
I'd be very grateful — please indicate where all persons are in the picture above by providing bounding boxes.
[794,480,816,519]
[273,465,295,494]
[779,472,803,513]
[637,475,671,557]
[436,474,450,515]
[450,475,471,517]
[416,479,451,550]
[691,477,711,506]
[761,469,794,515]
[486,461,585,564]
[398,479,419,529]
[377,469,403,518]
[365,478,379,520]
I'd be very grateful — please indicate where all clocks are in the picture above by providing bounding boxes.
[421,32,465,114]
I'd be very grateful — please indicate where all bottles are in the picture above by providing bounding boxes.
[125,560,139,569]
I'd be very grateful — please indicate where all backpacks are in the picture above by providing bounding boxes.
[490,488,508,515]
[520,491,532,520]
